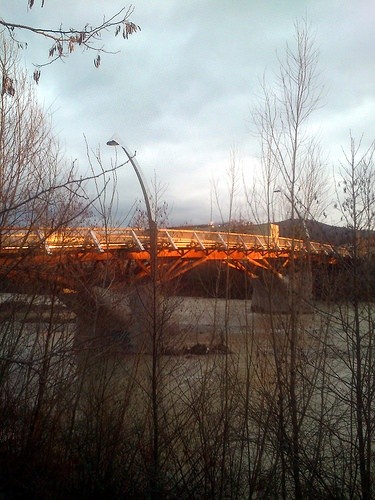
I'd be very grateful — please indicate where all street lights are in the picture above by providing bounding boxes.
[106,134,157,251]
[273,185,309,243]
